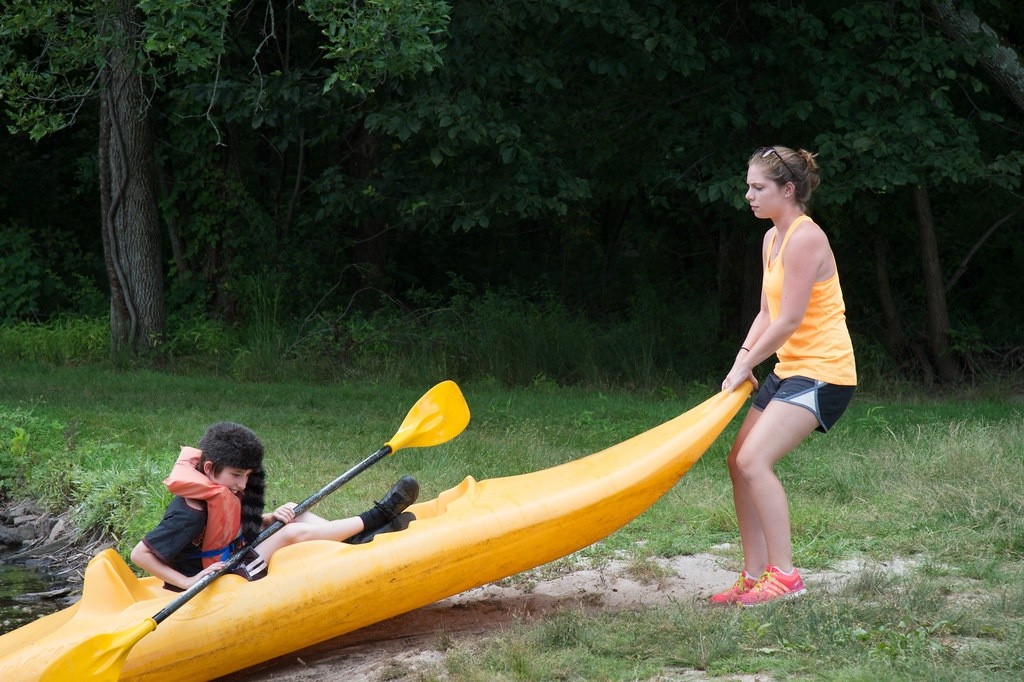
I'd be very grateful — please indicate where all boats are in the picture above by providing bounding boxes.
[0,381,757,682]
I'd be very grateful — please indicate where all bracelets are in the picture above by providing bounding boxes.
[740,347,749,352]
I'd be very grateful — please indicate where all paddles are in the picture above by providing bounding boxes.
[33,377,473,682]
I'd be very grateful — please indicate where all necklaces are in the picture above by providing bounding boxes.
[775,227,789,256]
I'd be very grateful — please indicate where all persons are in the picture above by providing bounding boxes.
[130,422,419,592]
[708,146,857,609]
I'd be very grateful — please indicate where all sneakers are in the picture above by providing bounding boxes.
[736,563,807,607]
[358,512,416,544]
[710,569,759,607]
[374,475,419,519]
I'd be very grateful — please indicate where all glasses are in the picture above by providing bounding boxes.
[752,146,795,179]
